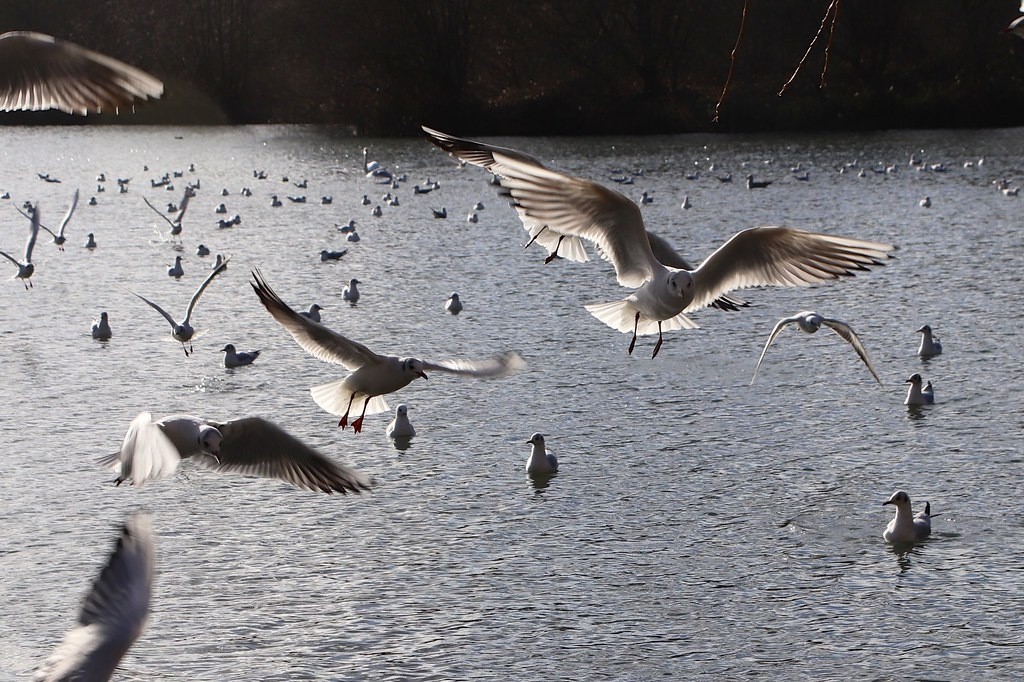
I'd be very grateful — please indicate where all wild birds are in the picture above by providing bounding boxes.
[119,253,234,357]
[909,148,1022,211]
[1,29,165,118]
[748,309,886,390]
[91,410,372,498]
[219,343,262,370]
[525,432,560,474]
[915,324,942,358]
[881,489,931,546]
[903,373,934,405]
[29,509,155,682]
[1,143,409,291]
[90,311,111,343]
[248,263,527,437]
[412,124,903,361]
